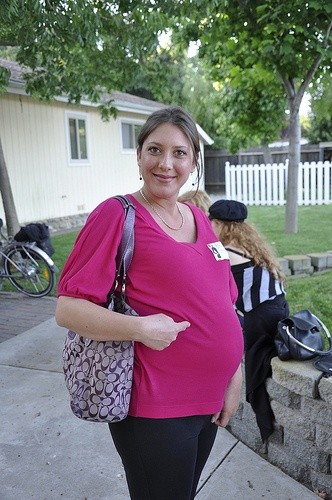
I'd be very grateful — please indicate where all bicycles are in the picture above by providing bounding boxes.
[0,217,58,299]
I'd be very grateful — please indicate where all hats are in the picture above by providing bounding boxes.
[208,200,248,221]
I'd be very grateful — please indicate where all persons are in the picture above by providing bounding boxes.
[56,108,244,500]
[208,200,290,350]
[179,190,213,216]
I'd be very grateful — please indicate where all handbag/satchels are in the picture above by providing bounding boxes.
[313,353,332,399]
[276,309,332,362]
[14,223,55,259]
[63,195,139,423]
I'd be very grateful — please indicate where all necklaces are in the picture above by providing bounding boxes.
[140,190,184,229]
